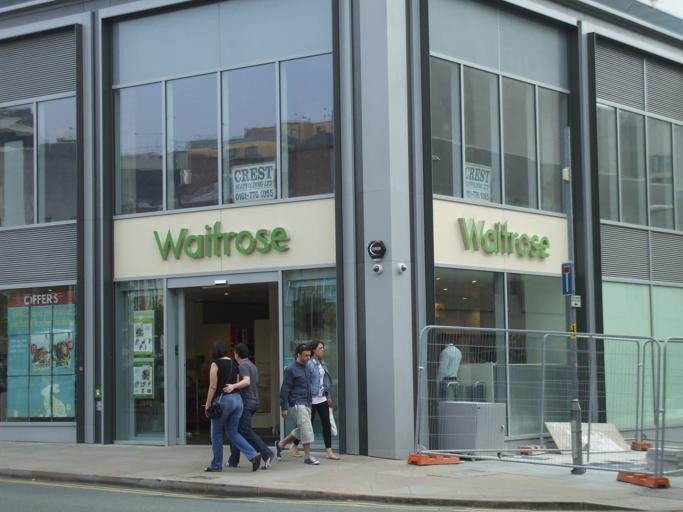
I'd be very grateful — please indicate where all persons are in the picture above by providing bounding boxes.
[436,335,462,381]
[203,340,341,472]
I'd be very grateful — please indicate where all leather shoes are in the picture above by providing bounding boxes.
[327,454,339,460]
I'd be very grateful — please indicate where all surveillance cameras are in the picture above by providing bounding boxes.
[372,264,383,274]
[399,263,407,273]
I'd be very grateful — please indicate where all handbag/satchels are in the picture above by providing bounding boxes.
[329,407,338,436]
[206,402,222,419]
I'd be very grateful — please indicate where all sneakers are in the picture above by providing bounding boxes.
[204,466,222,472]
[224,461,239,467]
[275,440,320,465]
[265,455,273,469]
[252,453,261,471]
